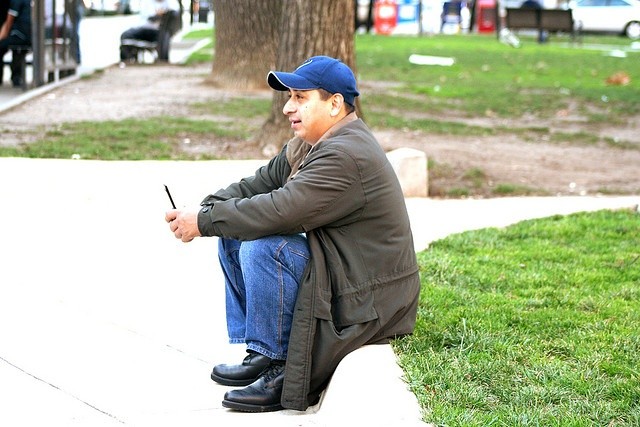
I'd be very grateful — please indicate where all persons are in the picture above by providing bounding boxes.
[1,0,31,89]
[163,57,422,412]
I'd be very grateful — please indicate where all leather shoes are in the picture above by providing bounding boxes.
[222,360,289,412]
[210,351,278,386]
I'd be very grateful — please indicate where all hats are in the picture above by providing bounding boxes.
[267,55,359,106]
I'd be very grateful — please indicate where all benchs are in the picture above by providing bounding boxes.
[118,9,176,62]
[504,6,584,43]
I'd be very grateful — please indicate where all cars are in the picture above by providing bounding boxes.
[555,0,638,40]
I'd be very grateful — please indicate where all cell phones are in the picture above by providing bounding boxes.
[165,184,177,211]
